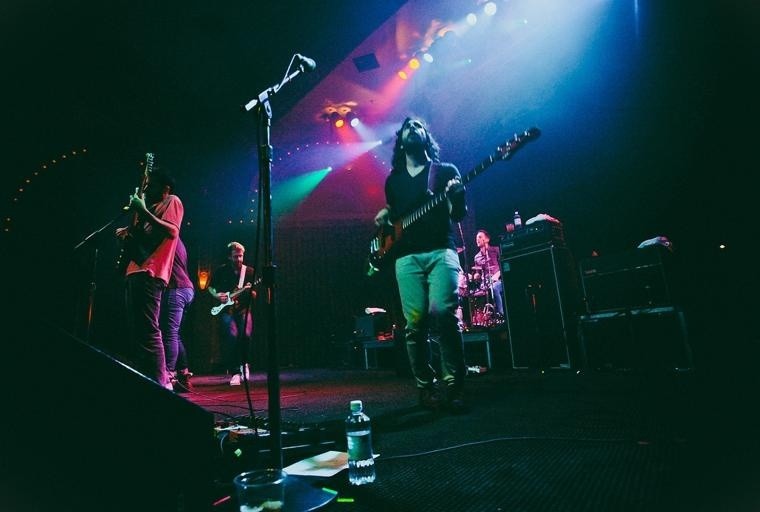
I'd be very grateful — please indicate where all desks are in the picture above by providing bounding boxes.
[362,334,506,369]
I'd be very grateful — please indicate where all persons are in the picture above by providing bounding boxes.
[112,165,185,392]
[163,236,195,379]
[444,214,472,335]
[466,227,504,323]
[372,114,470,416]
[206,241,260,386]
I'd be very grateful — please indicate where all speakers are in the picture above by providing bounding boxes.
[0,307,215,512]
[499,222,579,371]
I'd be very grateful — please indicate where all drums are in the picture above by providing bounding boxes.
[461,279,489,297]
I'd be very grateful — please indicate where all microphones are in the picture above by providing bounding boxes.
[525,284,537,315]
[296,53,316,72]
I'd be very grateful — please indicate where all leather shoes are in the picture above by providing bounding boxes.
[417,376,471,414]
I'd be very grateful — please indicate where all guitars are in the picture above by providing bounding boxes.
[112,151,154,269]
[210,276,263,319]
[366,124,542,270]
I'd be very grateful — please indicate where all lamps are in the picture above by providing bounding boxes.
[320,112,361,129]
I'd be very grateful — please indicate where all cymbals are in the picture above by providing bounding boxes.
[471,266,495,270]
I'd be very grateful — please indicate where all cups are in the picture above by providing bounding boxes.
[234,468,288,512]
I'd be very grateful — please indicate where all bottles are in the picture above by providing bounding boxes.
[344,400,376,486]
[514,211,522,230]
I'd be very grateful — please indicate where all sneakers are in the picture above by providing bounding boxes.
[165,373,193,393]
[230,363,250,387]
